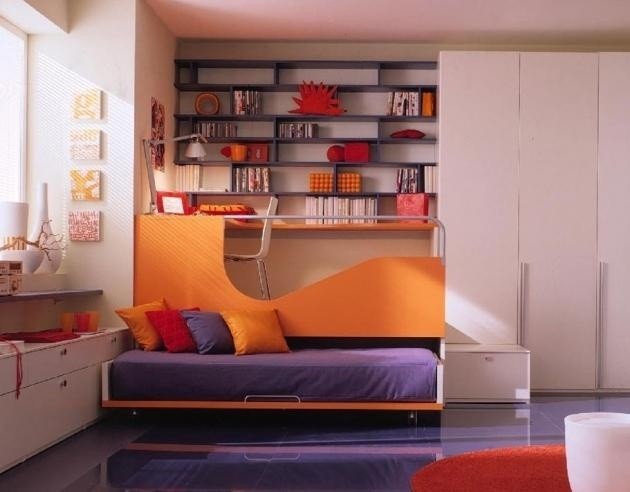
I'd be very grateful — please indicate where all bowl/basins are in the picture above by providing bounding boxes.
[230,144,247,162]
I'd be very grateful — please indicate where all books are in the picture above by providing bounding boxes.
[395,169,417,193]
[200,188,226,192]
[176,165,199,192]
[278,123,318,137]
[386,92,418,115]
[233,168,273,192]
[233,91,263,115]
[305,197,377,224]
[193,122,237,137]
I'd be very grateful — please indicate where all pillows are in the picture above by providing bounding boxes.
[219,307,292,356]
[115,297,166,351]
[144,307,203,353]
[179,308,236,354]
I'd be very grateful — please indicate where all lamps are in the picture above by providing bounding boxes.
[139,133,208,212]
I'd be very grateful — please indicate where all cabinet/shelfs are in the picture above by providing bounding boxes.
[0,327,132,476]
[440,342,531,404]
[437,49,598,395]
[171,58,440,257]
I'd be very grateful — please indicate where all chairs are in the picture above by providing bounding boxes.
[224,196,280,299]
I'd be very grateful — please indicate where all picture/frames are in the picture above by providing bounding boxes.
[151,95,167,173]
[67,210,100,241]
[69,171,100,201]
[71,89,101,121]
[154,192,189,215]
[69,129,101,161]
[196,94,219,114]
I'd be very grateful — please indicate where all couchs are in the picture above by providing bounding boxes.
[101,343,445,412]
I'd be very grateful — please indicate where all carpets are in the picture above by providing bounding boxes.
[409,444,573,492]
[599,51,630,395]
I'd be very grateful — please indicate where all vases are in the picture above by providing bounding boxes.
[0,201,45,272]
[28,182,62,273]
